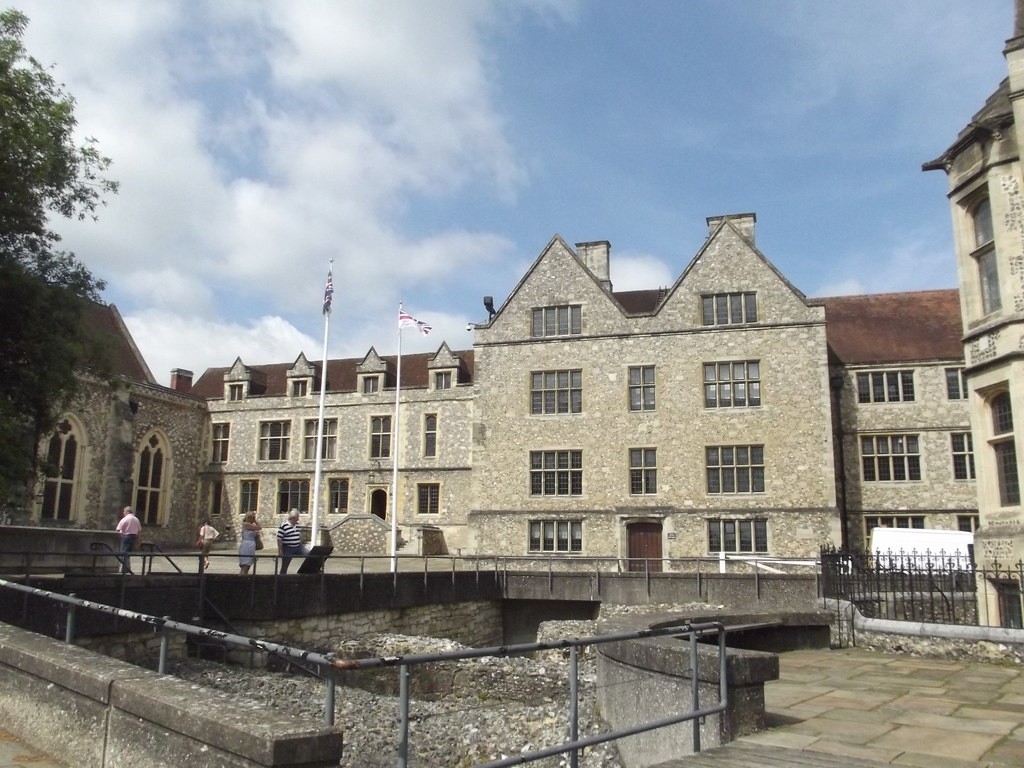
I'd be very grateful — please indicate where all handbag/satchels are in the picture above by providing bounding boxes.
[197,536,203,548]
[254,534,264,551]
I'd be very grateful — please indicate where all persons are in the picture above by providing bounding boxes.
[277,508,311,574]
[238,511,262,574]
[200,520,219,569]
[116,506,142,574]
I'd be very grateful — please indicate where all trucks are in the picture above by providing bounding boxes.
[869,526,974,576]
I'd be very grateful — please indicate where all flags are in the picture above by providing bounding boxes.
[399,310,431,336]
[322,270,333,314]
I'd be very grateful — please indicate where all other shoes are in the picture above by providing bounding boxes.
[204,562,209,569]
